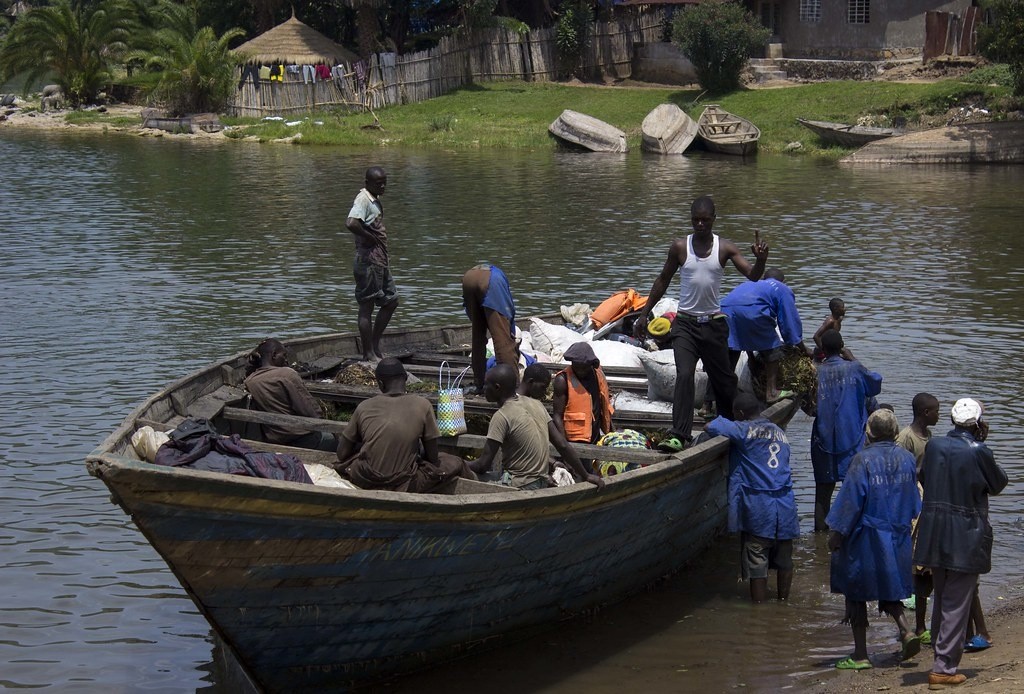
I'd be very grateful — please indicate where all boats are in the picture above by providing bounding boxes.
[547,109,630,153]
[698,104,761,155]
[641,102,699,154]
[795,116,907,146]
[85,307,803,693]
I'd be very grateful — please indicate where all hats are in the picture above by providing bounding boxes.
[951,398,981,425]
[563,342,600,369]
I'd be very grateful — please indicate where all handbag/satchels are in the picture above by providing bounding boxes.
[437,361,470,437]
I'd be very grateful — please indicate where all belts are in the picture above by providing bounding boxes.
[677,311,728,322]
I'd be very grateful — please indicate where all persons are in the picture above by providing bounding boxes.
[699,267,813,414]
[462,264,538,395]
[346,166,400,362]
[810,298,883,532]
[914,398,1011,690]
[824,393,939,668]
[703,392,800,604]
[336,357,478,494]
[463,363,605,494]
[635,196,769,452]
[553,341,614,443]
[246,338,338,452]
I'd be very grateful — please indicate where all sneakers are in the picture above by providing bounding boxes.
[928,672,966,689]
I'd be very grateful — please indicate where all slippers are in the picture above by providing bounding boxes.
[702,411,716,419]
[658,437,684,452]
[921,630,931,643]
[902,636,921,659]
[768,390,798,404]
[963,636,993,649]
[835,652,872,668]
